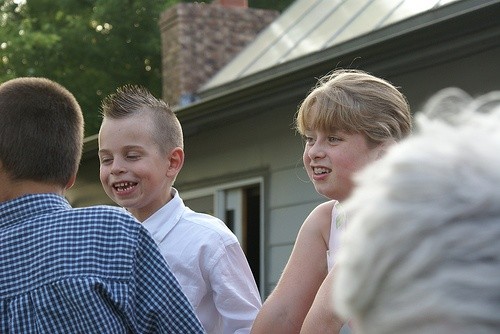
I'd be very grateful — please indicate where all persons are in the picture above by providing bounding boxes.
[97,84,263,334]
[328,86,500,334]
[248,68,413,334]
[0,76,207,334]
